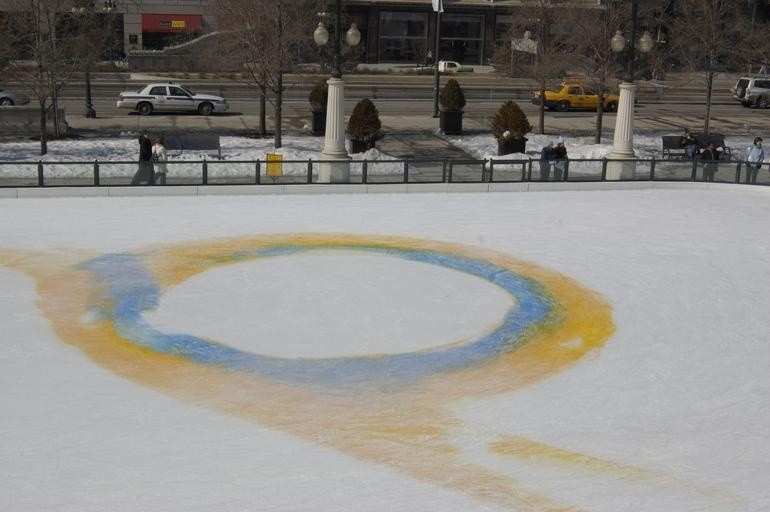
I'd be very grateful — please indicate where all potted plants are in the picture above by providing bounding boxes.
[347,98,385,153]
[488,98,531,155]
[438,79,465,134]
[309,80,327,137]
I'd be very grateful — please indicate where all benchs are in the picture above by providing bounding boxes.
[662,134,730,162]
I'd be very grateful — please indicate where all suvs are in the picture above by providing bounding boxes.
[729,76,770,108]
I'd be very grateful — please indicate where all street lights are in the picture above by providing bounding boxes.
[604,21,655,178]
[102,0,116,63]
[71,3,96,117]
[315,20,363,183]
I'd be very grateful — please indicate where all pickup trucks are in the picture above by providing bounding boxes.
[412,61,476,73]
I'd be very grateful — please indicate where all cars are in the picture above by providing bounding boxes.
[530,78,639,113]
[0,87,30,107]
[116,80,230,115]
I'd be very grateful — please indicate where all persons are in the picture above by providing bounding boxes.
[744,137,764,184]
[554,143,567,181]
[425,48,433,67]
[148,136,168,186]
[758,56,768,77]
[130,129,155,186]
[701,142,719,182]
[540,141,557,182]
